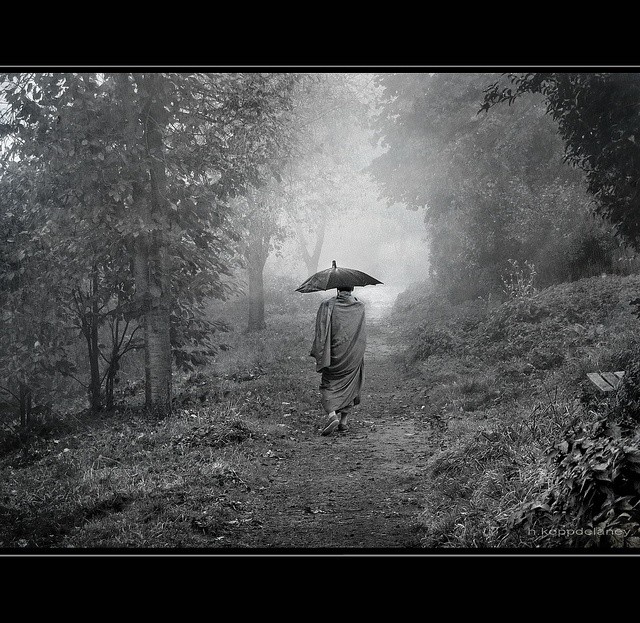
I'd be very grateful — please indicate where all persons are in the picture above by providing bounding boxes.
[308,287,367,436]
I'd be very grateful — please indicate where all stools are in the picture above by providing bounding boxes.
[586,370,624,394]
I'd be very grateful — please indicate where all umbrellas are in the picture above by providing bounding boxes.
[292,260,385,295]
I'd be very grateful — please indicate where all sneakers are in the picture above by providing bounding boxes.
[323,416,339,434]
[338,423,349,433]
